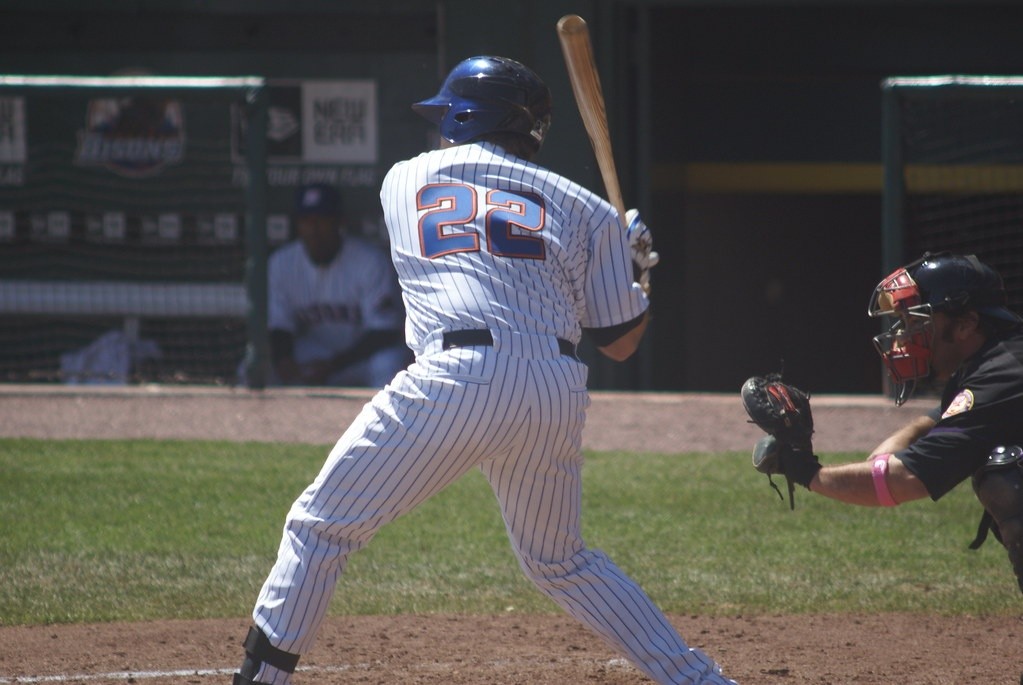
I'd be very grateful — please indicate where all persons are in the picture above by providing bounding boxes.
[242,182,407,387]
[232,56,738,685]
[743,254,1023,591]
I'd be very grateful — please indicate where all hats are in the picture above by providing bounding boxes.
[294,183,337,212]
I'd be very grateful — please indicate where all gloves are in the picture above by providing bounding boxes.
[624,208,659,284]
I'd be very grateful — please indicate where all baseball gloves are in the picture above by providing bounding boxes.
[738,373,818,488]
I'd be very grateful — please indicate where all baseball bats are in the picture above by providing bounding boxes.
[558,13,627,227]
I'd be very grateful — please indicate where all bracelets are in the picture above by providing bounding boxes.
[870,454,900,506]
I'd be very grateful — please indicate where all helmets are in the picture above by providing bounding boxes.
[907,251,1021,325]
[411,55,551,152]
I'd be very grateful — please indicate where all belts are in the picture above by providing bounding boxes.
[442,329,579,362]
